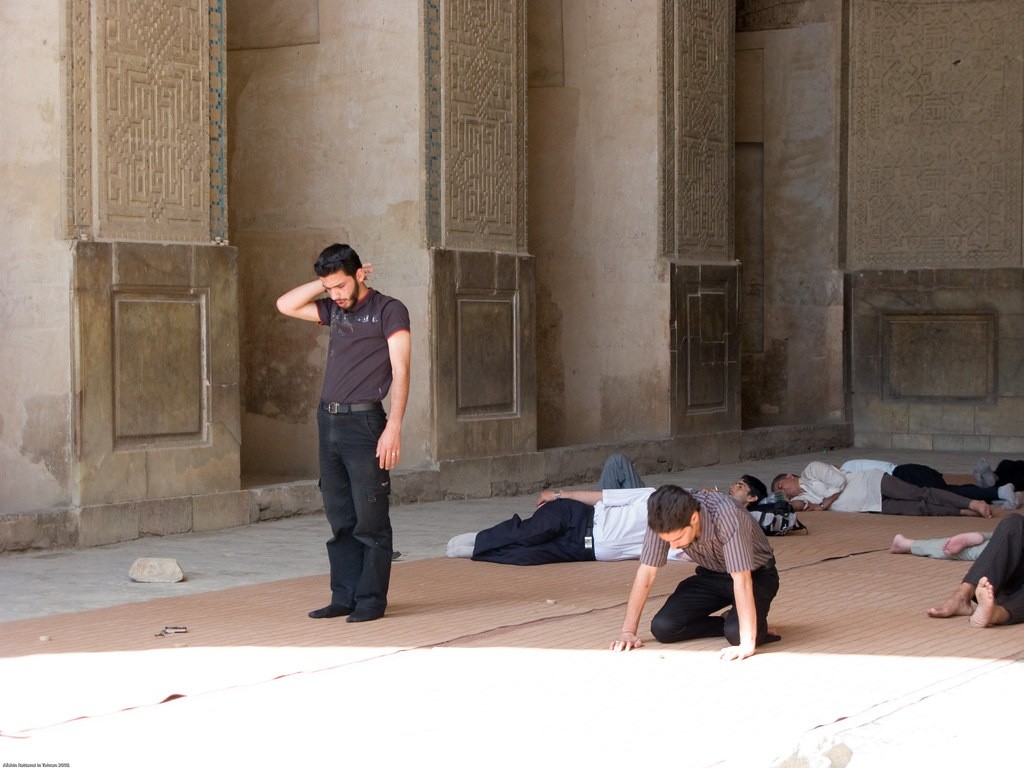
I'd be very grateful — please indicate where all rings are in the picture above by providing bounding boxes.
[616,641,619,642]
[392,453,396,456]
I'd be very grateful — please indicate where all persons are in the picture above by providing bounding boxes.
[890,513,1024,627]
[974,457,1024,493]
[276,243,411,622]
[833,459,1015,505]
[610,485,781,661]
[771,461,993,519]
[447,487,694,566]
[597,454,768,511]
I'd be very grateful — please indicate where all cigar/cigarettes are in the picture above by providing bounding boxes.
[715,486,717,490]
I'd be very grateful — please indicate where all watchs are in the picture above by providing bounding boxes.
[554,489,560,499]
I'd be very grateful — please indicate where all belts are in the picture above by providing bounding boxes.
[584,506,594,560]
[320,402,382,415]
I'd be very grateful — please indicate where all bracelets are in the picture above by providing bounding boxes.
[820,502,824,510]
